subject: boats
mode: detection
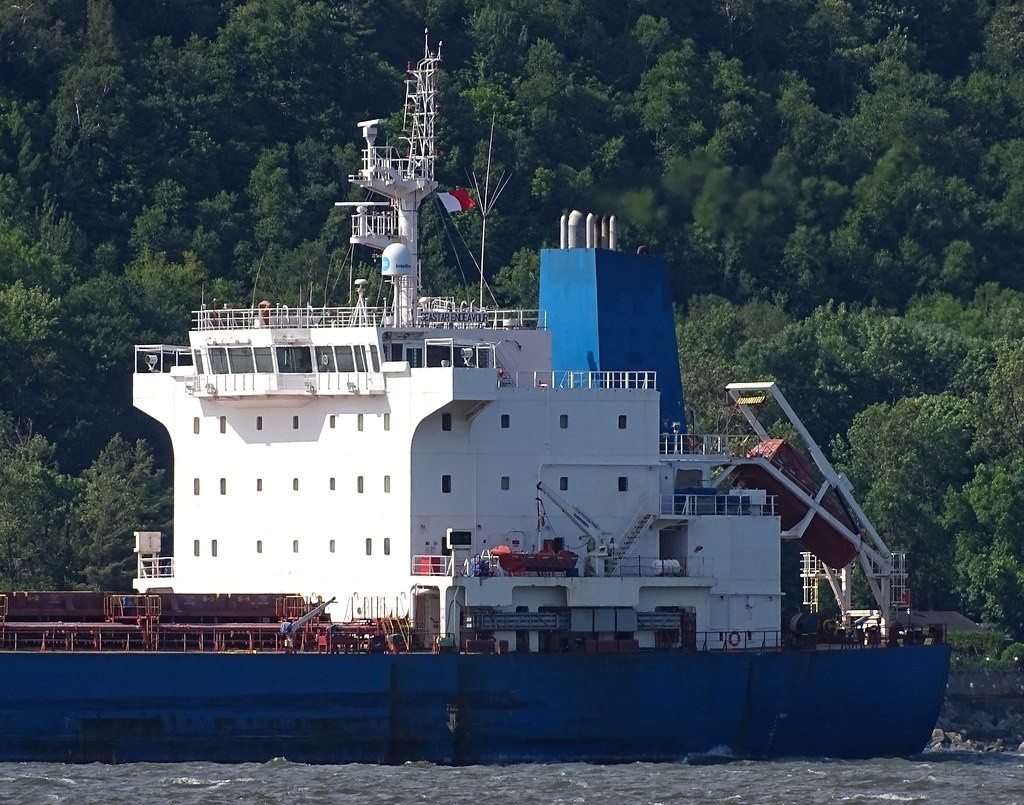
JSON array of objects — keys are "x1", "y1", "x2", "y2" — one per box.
[
  {"x1": 490, "y1": 546, "x2": 579, "y2": 573},
  {"x1": 740, "y1": 440, "x2": 862, "y2": 572},
  {"x1": 1, "y1": 28, "x2": 952, "y2": 768}
]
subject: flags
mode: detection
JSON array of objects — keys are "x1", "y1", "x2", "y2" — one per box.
[{"x1": 437, "y1": 190, "x2": 476, "y2": 213}]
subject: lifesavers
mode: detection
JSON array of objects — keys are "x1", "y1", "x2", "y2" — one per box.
[
  {"x1": 686, "y1": 435, "x2": 700, "y2": 448},
  {"x1": 729, "y1": 631, "x2": 740, "y2": 646},
  {"x1": 823, "y1": 619, "x2": 836, "y2": 634}
]
